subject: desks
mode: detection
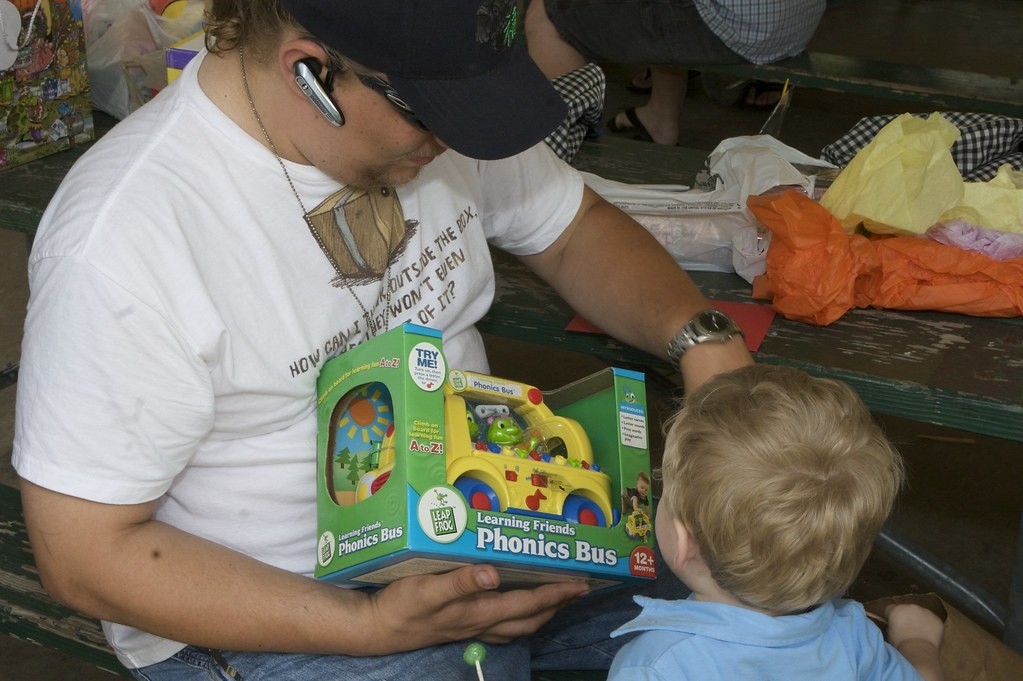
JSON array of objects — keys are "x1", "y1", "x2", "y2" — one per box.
[{"x1": 0, "y1": 129, "x2": 1022, "y2": 628}]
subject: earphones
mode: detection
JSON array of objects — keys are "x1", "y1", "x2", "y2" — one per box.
[{"x1": 293, "y1": 55, "x2": 345, "y2": 128}]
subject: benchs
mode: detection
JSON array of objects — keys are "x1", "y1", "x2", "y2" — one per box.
[
  {"x1": 0, "y1": 478, "x2": 132, "y2": 681},
  {"x1": 641, "y1": 51, "x2": 1022, "y2": 121}
]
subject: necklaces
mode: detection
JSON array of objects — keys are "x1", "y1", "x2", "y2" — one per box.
[{"x1": 237, "y1": 43, "x2": 398, "y2": 338}]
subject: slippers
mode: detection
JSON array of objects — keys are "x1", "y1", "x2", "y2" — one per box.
[
  {"x1": 732, "y1": 80, "x2": 791, "y2": 109},
  {"x1": 606, "y1": 107, "x2": 654, "y2": 143},
  {"x1": 639, "y1": 68, "x2": 652, "y2": 95}
]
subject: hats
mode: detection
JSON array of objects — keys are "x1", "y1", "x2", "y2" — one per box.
[{"x1": 286, "y1": 0, "x2": 568, "y2": 160}]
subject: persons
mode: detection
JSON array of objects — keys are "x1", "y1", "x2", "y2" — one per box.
[
  {"x1": 11, "y1": 0, "x2": 757, "y2": 679},
  {"x1": 522, "y1": 0, "x2": 826, "y2": 147},
  {"x1": 605, "y1": 363, "x2": 945, "y2": 681}
]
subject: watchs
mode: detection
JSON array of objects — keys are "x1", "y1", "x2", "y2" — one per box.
[{"x1": 666, "y1": 308, "x2": 748, "y2": 375}]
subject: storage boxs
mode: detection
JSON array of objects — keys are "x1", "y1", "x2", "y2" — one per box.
[
  {"x1": 0, "y1": 0, "x2": 93, "y2": 172},
  {"x1": 314, "y1": 322, "x2": 659, "y2": 591},
  {"x1": 164, "y1": 25, "x2": 210, "y2": 83}
]
